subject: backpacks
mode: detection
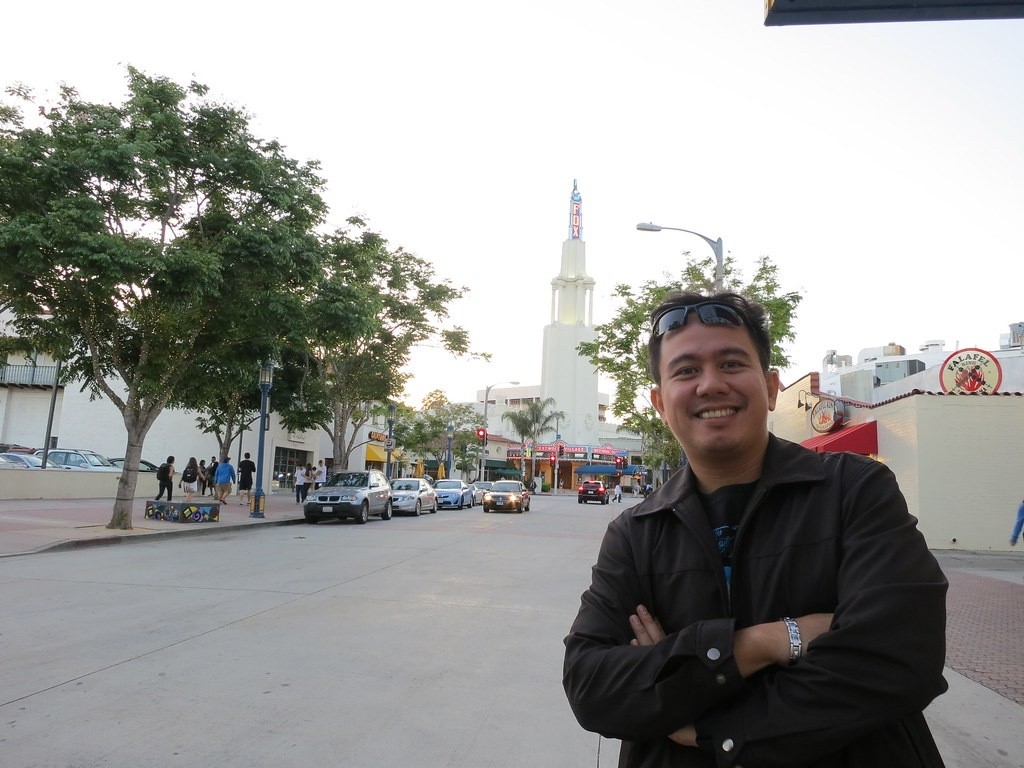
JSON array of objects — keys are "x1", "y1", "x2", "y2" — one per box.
[
  {"x1": 183, "y1": 466, "x2": 197, "y2": 483},
  {"x1": 157, "y1": 464, "x2": 171, "y2": 481}
]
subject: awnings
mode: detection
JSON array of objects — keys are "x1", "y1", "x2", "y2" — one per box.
[
  {"x1": 486, "y1": 460, "x2": 507, "y2": 467},
  {"x1": 366, "y1": 444, "x2": 394, "y2": 462},
  {"x1": 799, "y1": 420, "x2": 878, "y2": 454},
  {"x1": 415, "y1": 460, "x2": 447, "y2": 468}
]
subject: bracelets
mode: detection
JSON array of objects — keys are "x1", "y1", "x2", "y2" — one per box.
[{"x1": 780, "y1": 617, "x2": 802, "y2": 660}]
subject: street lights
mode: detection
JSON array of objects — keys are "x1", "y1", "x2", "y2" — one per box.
[
  {"x1": 480, "y1": 382, "x2": 521, "y2": 482},
  {"x1": 634, "y1": 222, "x2": 725, "y2": 294},
  {"x1": 248, "y1": 358, "x2": 273, "y2": 518},
  {"x1": 385, "y1": 405, "x2": 396, "y2": 480},
  {"x1": 446, "y1": 421, "x2": 454, "y2": 479}
]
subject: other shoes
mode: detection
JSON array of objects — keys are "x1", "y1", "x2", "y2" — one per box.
[{"x1": 221, "y1": 500, "x2": 227, "y2": 505}]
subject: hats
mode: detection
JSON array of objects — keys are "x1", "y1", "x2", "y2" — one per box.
[{"x1": 223, "y1": 457, "x2": 231, "y2": 463}]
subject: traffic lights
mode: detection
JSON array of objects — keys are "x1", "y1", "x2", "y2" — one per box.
[
  {"x1": 615, "y1": 457, "x2": 623, "y2": 470},
  {"x1": 617, "y1": 472, "x2": 621, "y2": 477},
  {"x1": 549, "y1": 454, "x2": 556, "y2": 467},
  {"x1": 621, "y1": 457, "x2": 629, "y2": 469},
  {"x1": 559, "y1": 445, "x2": 564, "y2": 456},
  {"x1": 558, "y1": 469, "x2": 562, "y2": 474},
  {"x1": 477, "y1": 428, "x2": 488, "y2": 446}
]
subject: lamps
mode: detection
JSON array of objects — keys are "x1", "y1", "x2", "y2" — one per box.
[
  {"x1": 804, "y1": 392, "x2": 818, "y2": 411},
  {"x1": 797, "y1": 390, "x2": 814, "y2": 408}
]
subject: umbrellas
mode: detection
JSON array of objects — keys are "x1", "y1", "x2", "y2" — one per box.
[
  {"x1": 437, "y1": 462, "x2": 445, "y2": 480},
  {"x1": 414, "y1": 457, "x2": 425, "y2": 478},
  {"x1": 495, "y1": 468, "x2": 520, "y2": 480}
]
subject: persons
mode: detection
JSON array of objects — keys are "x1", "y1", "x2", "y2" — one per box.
[
  {"x1": 295, "y1": 460, "x2": 326, "y2": 505},
  {"x1": 1009, "y1": 501, "x2": 1024, "y2": 546},
  {"x1": 178, "y1": 457, "x2": 206, "y2": 501},
  {"x1": 238, "y1": 452, "x2": 256, "y2": 506},
  {"x1": 633, "y1": 482, "x2": 641, "y2": 498},
  {"x1": 198, "y1": 457, "x2": 219, "y2": 496},
  {"x1": 214, "y1": 457, "x2": 236, "y2": 506},
  {"x1": 155, "y1": 456, "x2": 175, "y2": 501},
  {"x1": 563, "y1": 291, "x2": 949, "y2": 768},
  {"x1": 612, "y1": 482, "x2": 622, "y2": 503},
  {"x1": 646, "y1": 483, "x2": 652, "y2": 491}
]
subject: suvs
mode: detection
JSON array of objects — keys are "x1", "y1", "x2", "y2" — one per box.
[
  {"x1": 302, "y1": 467, "x2": 393, "y2": 524},
  {"x1": 577, "y1": 480, "x2": 610, "y2": 505}
]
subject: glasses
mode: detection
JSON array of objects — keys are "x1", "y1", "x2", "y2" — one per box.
[{"x1": 653, "y1": 301, "x2": 759, "y2": 342}]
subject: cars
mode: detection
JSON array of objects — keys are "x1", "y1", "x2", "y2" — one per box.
[
  {"x1": 431, "y1": 479, "x2": 473, "y2": 510},
  {"x1": 482, "y1": 479, "x2": 531, "y2": 514},
  {"x1": 0, "y1": 442, "x2": 123, "y2": 471},
  {"x1": 108, "y1": 457, "x2": 159, "y2": 472},
  {"x1": 388, "y1": 477, "x2": 438, "y2": 516},
  {"x1": 469, "y1": 481, "x2": 495, "y2": 506}
]
